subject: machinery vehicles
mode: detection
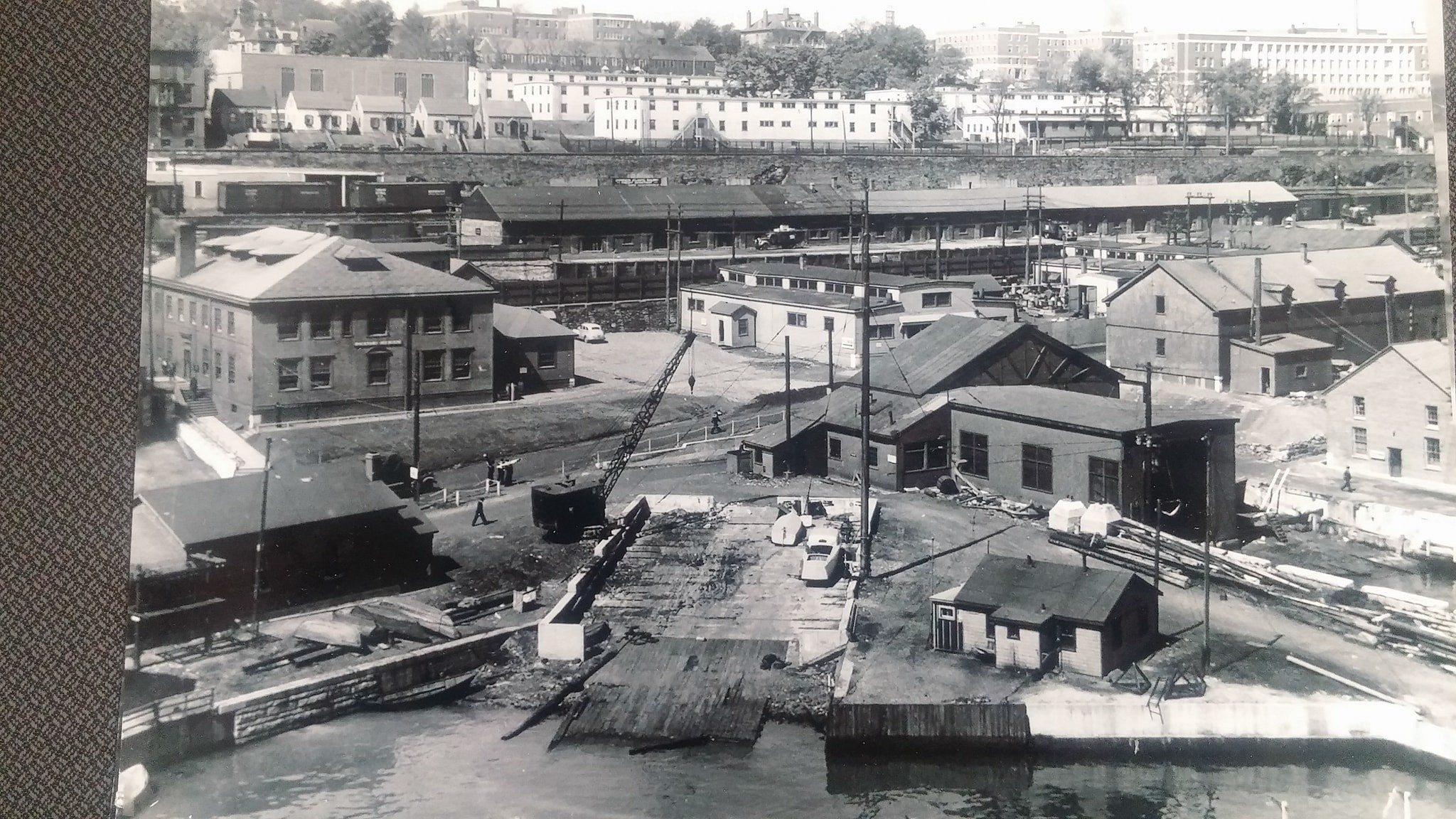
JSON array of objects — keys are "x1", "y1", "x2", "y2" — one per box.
[{"x1": 531, "y1": 330, "x2": 697, "y2": 535}]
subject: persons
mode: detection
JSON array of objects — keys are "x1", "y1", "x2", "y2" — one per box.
[
  {"x1": 1341, "y1": 467, "x2": 1352, "y2": 492},
  {"x1": 472, "y1": 497, "x2": 489, "y2": 526},
  {"x1": 711, "y1": 411, "x2": 722, "y2": 434},
  {"x1": 486, "y1": 459, "x2": 513, "y2": 487}
]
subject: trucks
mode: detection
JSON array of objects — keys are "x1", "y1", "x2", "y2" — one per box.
[
  {"x1": 754, "y1": 229, "x2": 806, "y2": 250},
  {"x1": 799, "y1": 527, "x2": 843, "y2": 580},
  {"x1": 379, "y1": 453, "x2": 437, "y2": 497}
]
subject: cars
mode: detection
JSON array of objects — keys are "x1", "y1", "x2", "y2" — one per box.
[{"x1": 572, "y1": 322, "x2": 605, "y2": 343}]
